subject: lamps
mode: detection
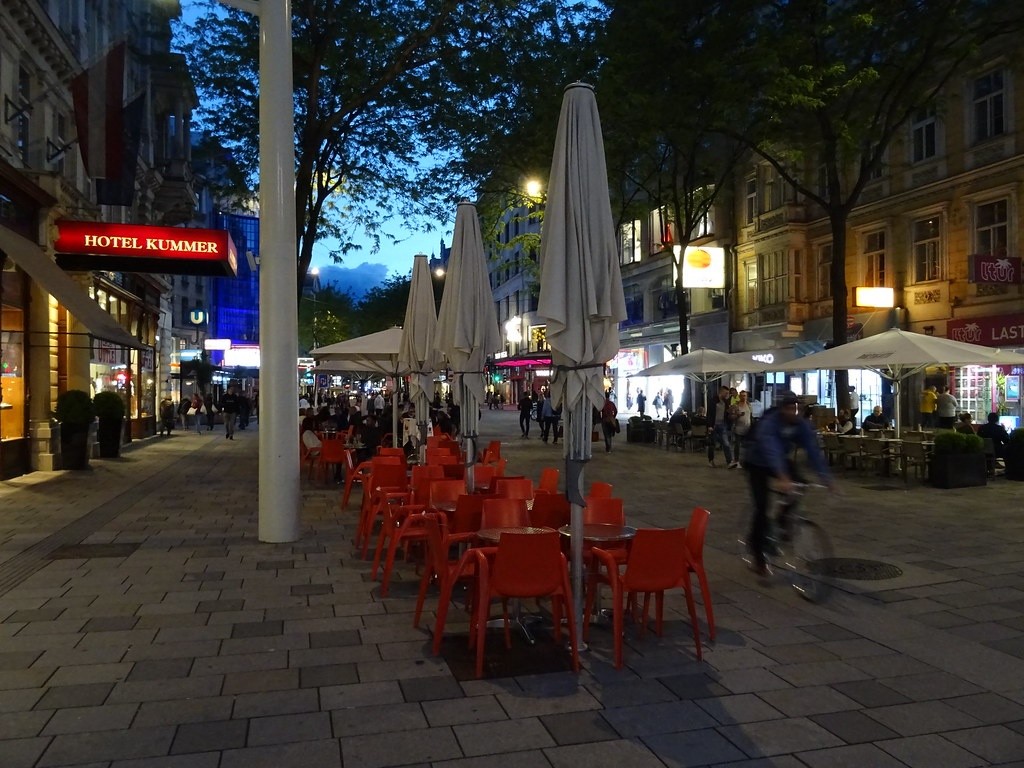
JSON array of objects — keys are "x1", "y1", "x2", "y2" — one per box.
[{"x1": 852, "y1": 286, "x2": 894, "y2": 308}]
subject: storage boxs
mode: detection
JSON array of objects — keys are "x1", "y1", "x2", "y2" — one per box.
[{"x1": 798, "y1": 395, "x2": 835, "y2": 427}]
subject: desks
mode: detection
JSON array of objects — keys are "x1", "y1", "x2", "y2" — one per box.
[
  {"x1": 869, "y1": 428, "x2": 895, "y2": 438},
  {"x1": 524, "y1": 499, "x2": 535, "y2": 510},
  {"x1": 816, "y1": 431, "x2": 843, "y2": 468},
  {"x1": 555, "y1": 522, "x2": 636, "y2": 634},
  {"x1": 903, "y1": 439, "x2": 935, "y2": 485},
  {"x1": 875, "y1": 437, "x2": 903, "y2": 479},
  {"x1": 908, "y1": 430, "x2": 934, "y2": 439},
  {"x1": 476, "y1": 529, "x2": 554, "y2": 645},
  {"x1": 430, "y1": 499, "x2": 469, "y2": 591},
  {"x1": 839, "y1": 435, "x2": 868, "y2": 472}
]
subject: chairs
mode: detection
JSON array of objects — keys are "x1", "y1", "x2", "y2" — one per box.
[
  {"x1": 804, "y1": 427, "x2": 1006, "y2": 485},
  {"x1": 653, "y1": 419, "x2": 708, "y2": 453},
  {"x1": 301, "y1": 430, "x2": 715, "y2": 679}
]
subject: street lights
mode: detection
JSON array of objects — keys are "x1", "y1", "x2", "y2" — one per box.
[{"x1": 311, "y1": 267, "x2": 320, "y2": 351}]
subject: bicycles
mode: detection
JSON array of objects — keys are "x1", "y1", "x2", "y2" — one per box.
[{"x1": 738, "y1": 482, "x2": 834, "y2": 603}]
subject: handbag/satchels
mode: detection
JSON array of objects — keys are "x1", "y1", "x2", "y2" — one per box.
[
  {"x1": 187, "y1": 407, "x2": 196, "y2": 415},
  {"x1": 201, "y1": 404, "x2": 207, "y2": 415},
  {"x1": 211, "y1": 403, "x2": 218, "y2": 413},
  {"x1": 606, "y1": 415, "x2": 617, "y2": 428}
]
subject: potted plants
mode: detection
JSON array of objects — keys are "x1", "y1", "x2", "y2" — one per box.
[
  {"x1": 626, "y1": 415, "x2": 655, "y2": 443},
  {"x1": 1002, "y1": 428, "x2": 1024, "y2": 481},
  {"x1": 928, "y1": 428, "x2": 988, "y2": 488},
  {"x1": 93, "y1": 390, "x2": 126, "y2": 459},
  {"x1": 56, "y1": 390, "x2": 96, "y2": 469}
]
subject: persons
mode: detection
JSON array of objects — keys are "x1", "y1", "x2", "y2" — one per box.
[
  {"x1": 190, "y1": 383, "x2": 260, "y2": 441},
  {"x1": 299, "y1": 385, "x2": 499, "y2": 485},
  {"x1": 516, "y1": 384, "x2": 1008, "y2": 567}
]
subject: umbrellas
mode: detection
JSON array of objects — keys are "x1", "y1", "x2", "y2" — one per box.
[
  {"x1": 625, "y1": 346, "x2": 773, "y2": 417},
  {"x1": 398, "y1": 254, "x2": 448, "y2": 446},
  {"x1": 770, "y1": 327, "x2": 1024, "y2": 471},
  {"x1": 307, "y1": 323, "x2": 451, "y2": 450},
  {"x1": 431, "y1": 198, "x2": 504, "y2": 492},
  {"x1": 308, "y1": 360, "x2": 398, "y2": 379},
  {"x1": 534, "y1": 79, "x2": 628, "y2": 653}
]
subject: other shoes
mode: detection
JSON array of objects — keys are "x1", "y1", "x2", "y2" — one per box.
[
  {"x1": 552, "y1": 441, "x2": 557, "y2": 444},
  {"x1": 226, "y1": 433, "x2": 230, "y2": 439},
  {"x1": 230, "y1": 436, "x2": 233, "y2": 440},
  {"x1": 207, "y1": 427, "x2": 210, "y2": 431},
  {"x1": 726, "y1": 463, "x2": 737, "y2": 469},
  {"x1": 709, "y1": 461, "x2": 716, "y2": 468},
  {"x1": 211, "y1": 427, "x2": 214, "y2": 430},
  {"x1": 751, "y1": 553, "x2": 774, "y2": 578},
  {"x1": 543, "y1": 438, "x2": 546, "y2": 442},
  {"x1": 522, "y1": 433, "x2": 527, "y2": 440}
]
